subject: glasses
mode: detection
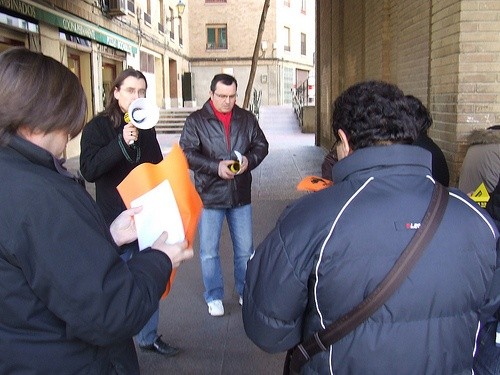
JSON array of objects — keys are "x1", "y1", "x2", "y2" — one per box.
[
  {"x1": 329, "y1": 139, "x2": 339, "y2": 158},
  {"x1": 214, "y1": 91, "x2": 238, "y2": 101},
  {"x1": 119, "y1": 87, "x2": 146, "y2": 96}
]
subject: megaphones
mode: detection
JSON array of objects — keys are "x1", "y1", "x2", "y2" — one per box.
[{"x1": 123, "y1": 98, "x2": 160, "y2": 147}]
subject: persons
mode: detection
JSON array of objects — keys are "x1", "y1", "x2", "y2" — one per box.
[
  {"x1": 79, "y1": 67, "x2": 183, "y2": 355},
  {"x1": 0, "y1": 46, "x2": 194, "y2": 375},
  {"x1": 179, "y1": 74, "x2": 269, "y2": 316},
  {"x1": 243, "y1": 81, "x2": 499, "y2": 375}
]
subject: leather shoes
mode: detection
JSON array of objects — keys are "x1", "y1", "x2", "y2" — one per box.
[{"x1": 139, "y1": 335, "x2": 180, "y2": 357}]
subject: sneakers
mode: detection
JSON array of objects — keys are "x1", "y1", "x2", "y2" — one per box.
[
  {"x1": 207, "y1": 299, "x2": 224, "y2": 316},
  {"x1": 238, "y1": 297, "x2": 243, "y2": 306}
]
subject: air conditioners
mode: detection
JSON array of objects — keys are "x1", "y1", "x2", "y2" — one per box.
[{"x1": 108, "y1": 0, "x2": 128, "y2": 15}]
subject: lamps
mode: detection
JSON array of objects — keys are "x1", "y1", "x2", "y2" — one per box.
[{"x1": 166, "y1": 0, "x2": 185, "y2": 24}]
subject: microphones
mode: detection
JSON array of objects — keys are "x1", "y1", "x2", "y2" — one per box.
[{"x1": 231, "y1": 162, "x2": 240, "y2": 174}]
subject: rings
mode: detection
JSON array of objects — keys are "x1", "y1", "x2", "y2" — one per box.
[{"x1": 131, "y1": 131, "x2": 133, "y2": 136}]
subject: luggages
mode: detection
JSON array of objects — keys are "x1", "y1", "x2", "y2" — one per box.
[{"x1": 322, "y1": 154, "x2": 338, "y2": 181}]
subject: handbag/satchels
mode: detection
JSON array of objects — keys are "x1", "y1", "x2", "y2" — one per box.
[{"x1": 283, "y1": 349, "x2": 305, "y2": 375}]
